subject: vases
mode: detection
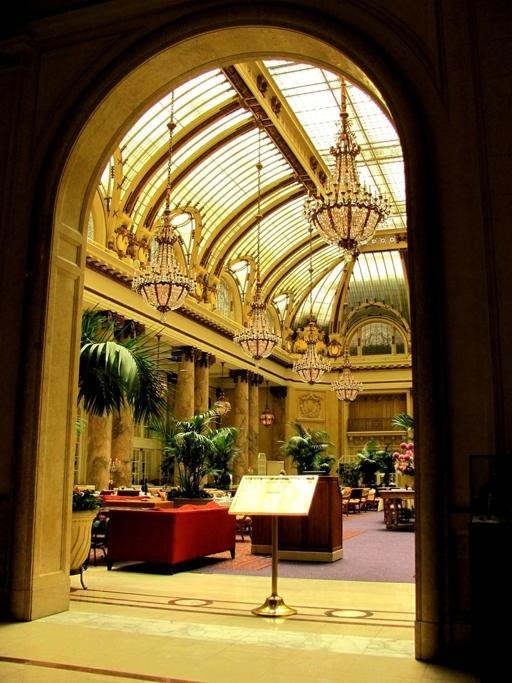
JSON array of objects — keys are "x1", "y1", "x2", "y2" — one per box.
[
  {"x1": 70, "y1": 507, "x2": 100, "y2": 571},
  {"x1": 398, "y1": 475, "x2": 415, "y2": 490}
]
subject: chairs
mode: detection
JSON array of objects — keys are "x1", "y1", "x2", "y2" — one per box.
[{"x1": 97, "y1": 486, "x2": 379, "y2": 576}]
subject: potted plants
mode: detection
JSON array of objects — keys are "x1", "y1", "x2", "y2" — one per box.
[{"x1": 144, "y1": 405, "x2": 240, "y2": 506}]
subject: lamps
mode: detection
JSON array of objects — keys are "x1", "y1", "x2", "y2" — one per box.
[
  {"x1": 150, "y1": 334, "x2": 168, "y2": 402},
  {"x1": 330, "y1": 277, "x2": 362, "y2": 402},
  {"x1": 260, "y1": 381, "x2": 275, "y2": 429},
  {"x1": 131, "y1": 90, "x2": 195, "y2": 313},
  {"x1": 292, "y1": 192, "x2": 329, "y2": 384},
  {"x1": 214, "y1": 361, "x2": 231, "y2": 417},
  {"x1": 233, "y1": 128, "x2": 283, "y2": 359},
  {"x1": 301, "y1": 76, "x2": 391, "y2": 262}
]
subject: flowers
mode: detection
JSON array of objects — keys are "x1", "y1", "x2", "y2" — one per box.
[
  {"x1": 72, "y1": 485, "x2": 101, "y2": 512},
  {"x1": 391, "y1": 442, "x2": 416, "y2": 475}
]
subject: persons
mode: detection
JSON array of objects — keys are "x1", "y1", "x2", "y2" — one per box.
[
  {"x1": 341, "y1": 469, "x2": 396, "y2": 510},
  {"x1": 279, "y1": 469, "x2": 286, "y2": 475}
]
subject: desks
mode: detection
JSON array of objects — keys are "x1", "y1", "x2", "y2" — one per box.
[{"x1": 378, "y1": 490, "x2": 416, "y2": 531}]
